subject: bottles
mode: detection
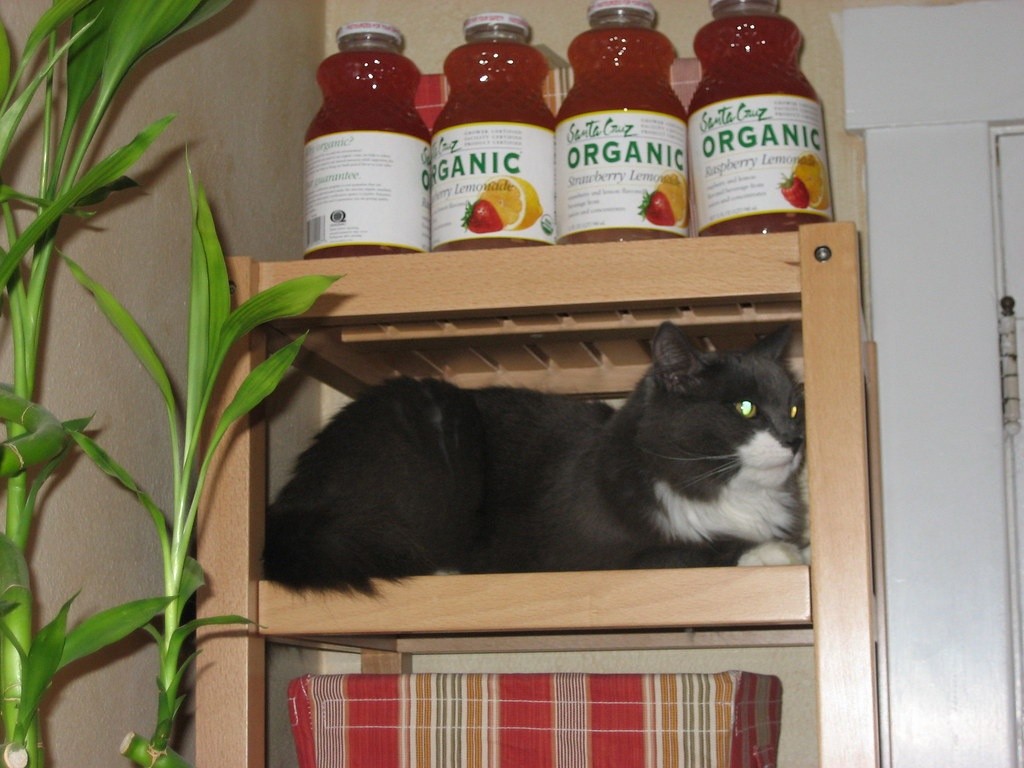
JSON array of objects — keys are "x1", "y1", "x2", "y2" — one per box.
[
  {"x1": 300, "y1": 21, "x2": 431, "y2": 262},
  {"x1": 687, "y1": 0, "x2": 837, "y2": 238},
  {"x1": 430, "y1": 9, "x2": 554, "y2": 251},
  {"x1": 555, "y1": 1, "x2": 690, "y2": 241}
]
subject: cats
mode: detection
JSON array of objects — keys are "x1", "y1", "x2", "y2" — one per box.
[{"x1": 260, "y1": 319, "x2": 813, "y2": 601}]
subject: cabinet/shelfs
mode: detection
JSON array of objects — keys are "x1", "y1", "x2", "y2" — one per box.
[{"x1": 190, "y1": 222, "x2": 881, "y2": 768}]
